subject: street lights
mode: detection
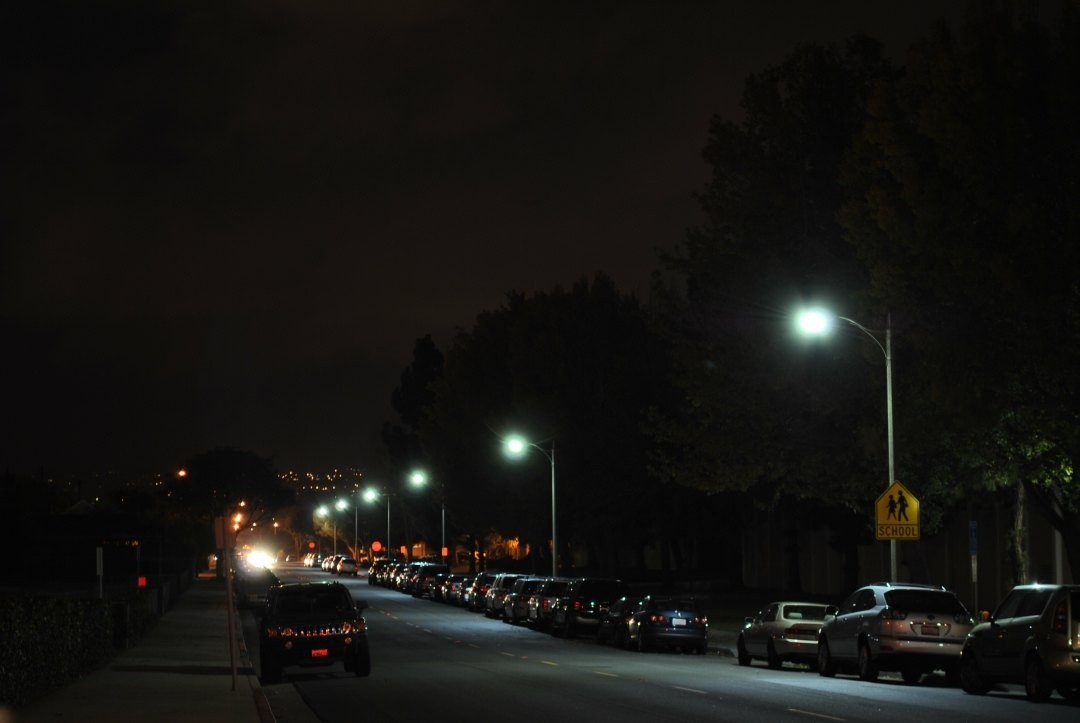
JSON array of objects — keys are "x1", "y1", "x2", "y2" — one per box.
[
  {"x1": 366, "y1": 491, "x2": 391, "y2": 559},
  {"x1": 340, "y1": 500, "x2": 358, "y2": 555},
  {"x1": 509, "y1": 438, "x2": 559, "y2": 578},
  {"x1": 414, "y1": 474, "x2": 447, "y2": 565},
  {"x1": 799, "y1": 306, "x2": 898, "y2": 581},
  {"x1": 318, "y1": 504, "x2": 337, "y2": 556}
]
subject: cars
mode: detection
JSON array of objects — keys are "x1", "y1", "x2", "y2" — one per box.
[
  {"x1": 959, "y1": 582, "x2": 1080, "y2": 705},
  {"x1": 736, "y1": 602, "x2": 839, "y2": 669},
  {"x1": 231, "y1": 557, "x2": 283, "y2": 606},
  {"x1": 302, "y1": 552, "x2": 358, "y2": 577},
  {"x1": 260, "y1": 581, "x2": 370, "y2": 672},
  {"x1": 367, "y1": 559, "x2": 710, "y2": 656}
]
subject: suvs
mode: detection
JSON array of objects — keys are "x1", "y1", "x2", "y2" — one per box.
[{"x1": 817, "y1": 581, "x2": 978, "y2": 684}]
